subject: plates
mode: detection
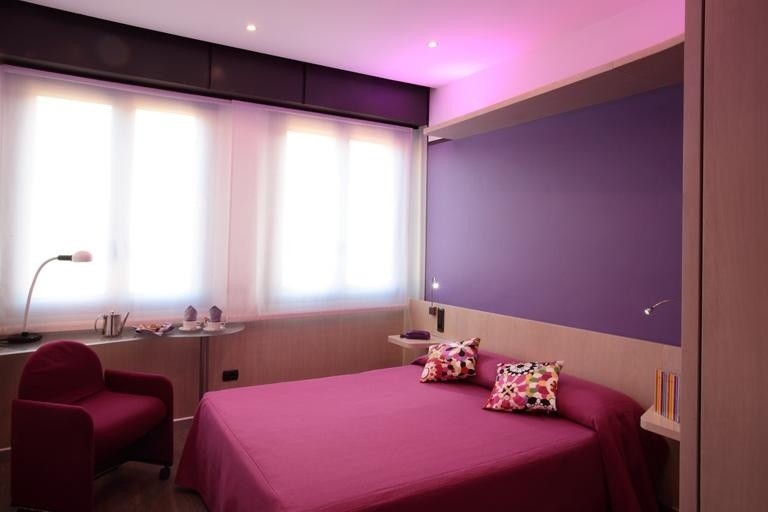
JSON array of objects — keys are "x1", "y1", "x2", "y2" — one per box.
[
  {"x1": 178, "y1": 326, "x2": 201, "y2": 331},
  {"x1": 130, "y1": 321, "x2": 174, "y2": 334},
  {"x1": 203, "y1": 326, "x2": 226, "y2": 331}
]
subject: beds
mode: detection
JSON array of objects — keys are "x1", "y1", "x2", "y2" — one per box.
[{"x1": 193, "y1": 344, "x2": 656, "y2": 511}]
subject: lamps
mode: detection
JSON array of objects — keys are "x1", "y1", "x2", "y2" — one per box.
[
  {"x1": 6, "y1": 250, "x2": 94, "y2": 345},
  {"x1": 428, "y1": 276, "x2": 440, "y2": 316},
  {"x1": 643, "y1": 297, "x2": 681, "y2": 316}
]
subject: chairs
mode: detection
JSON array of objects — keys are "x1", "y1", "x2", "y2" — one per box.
[{"x1": 11, "y1": 340, "x2": 174, "y2": 512}]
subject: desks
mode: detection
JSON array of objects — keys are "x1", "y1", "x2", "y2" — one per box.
[
  {"x1": 387, "y1": 334, "x2": 440, "y2": 366},
  {"x1": 640, "y1": 402, "x2": 680, "y2": 511},
  {"x1": 0, "y1": 319, "x2": 246, "y2": 402}
]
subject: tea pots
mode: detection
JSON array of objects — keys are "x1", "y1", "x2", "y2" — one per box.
[{"x1": 94, "y1": 311, "x2": 130, "y2": 336}]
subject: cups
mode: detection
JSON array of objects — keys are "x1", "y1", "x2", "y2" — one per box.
[
  {"x1": 183, "y1": 321, "x2": 201, "y2": 330},
  {"x1": 207, "y1": 321, "x2": 225, "y2": 329}
]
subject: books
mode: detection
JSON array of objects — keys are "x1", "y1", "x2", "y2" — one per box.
[{"x1": 655, "y1": 369, "x2": 680, "y2": 423}]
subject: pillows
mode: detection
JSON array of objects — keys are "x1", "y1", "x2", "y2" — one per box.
[{"x1": 419, "y1": 335, "x2": 564, "y2": 414}]
